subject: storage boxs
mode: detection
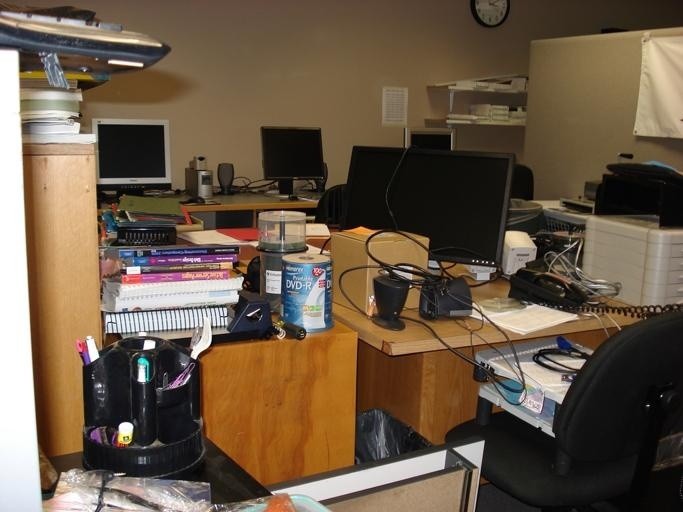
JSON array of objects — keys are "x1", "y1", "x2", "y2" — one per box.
[{"x1": 329, "y1": 225, "x2": 430, "y2": 318}]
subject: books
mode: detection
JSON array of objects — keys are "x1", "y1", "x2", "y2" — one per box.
[
  {"x1": 15, "y1": 66, "x2": 111, "y2": 146},
  {"x1": 471, "y1": 337, "x2": 601, "y2": 438},
  {"x1": 91, "y1": 194, "x2": 261, "y2": 340},
  {"x1": 275, "y1": 222, "x2": 330, "y2": 241}
]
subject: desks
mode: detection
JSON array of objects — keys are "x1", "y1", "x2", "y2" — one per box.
[
  {"x1": 40, "y1": 435, "x2": 275, "y2": 511},
  {"x1": 159, "y1": 190, "x2": 319, "y2": 229},
  {"x1": 177, "y1": 227, "x2": 648, "y2": 446}
]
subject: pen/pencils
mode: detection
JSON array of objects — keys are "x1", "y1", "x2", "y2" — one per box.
[
  {"x1": 76, "y1": 335, "x2": 100, "y2": 366},
  {"x1": 136, "y1": 358, "x2": 150, "y2": 383}
]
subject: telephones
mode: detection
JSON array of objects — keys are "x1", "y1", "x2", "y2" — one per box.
[{"x1": 508, "y1": 267, "x2": 594, "y2": 314}]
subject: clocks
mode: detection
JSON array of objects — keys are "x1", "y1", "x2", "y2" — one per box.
[{"x1": 467, "y1": 0, "x2": 512, "y2": 29}]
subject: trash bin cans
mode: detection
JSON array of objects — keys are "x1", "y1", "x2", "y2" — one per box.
[{"x1": 355, "y1": 407, "x2": 433, "y2": 465}]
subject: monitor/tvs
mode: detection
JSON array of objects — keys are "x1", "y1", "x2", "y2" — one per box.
[
  {"x1": 340, "y1": 145, "x2": 516, "y2": 267},
  {"x1": 403, "y1": 127, "x2": 456, "y2": 151},
  {"x1": 260, "y1": 125, "x2": 325, "y2": 199},
  {"x1": 92, "y1": 117, "x2": 172, "y2": 197}
]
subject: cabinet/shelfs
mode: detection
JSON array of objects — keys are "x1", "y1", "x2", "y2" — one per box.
[
  {"x1": 445, "y1": 85, "x2": 528, "y2": 126},
  {"x1": 194, "y1": 317, "x2": 358, "y2": 487},
  {"x1": 21, "y1": 141, "x2": 107, "y2": 456}
]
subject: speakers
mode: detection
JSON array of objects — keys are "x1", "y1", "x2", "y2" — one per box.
[
  {"x1": 217, "y1": 163, "x2": 235, "y2": 195},
  {"x1": 185, "y1": 168, "x2": 213, "y2": 198},
  {"x1": 308, "y1": 163, "x2": 330, "y2": 192}
]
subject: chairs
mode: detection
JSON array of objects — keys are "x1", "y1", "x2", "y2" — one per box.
[
  {"x1": 509, "y1": 163, "x2": 535, "y2": 201},
  {"x1": 442, "y1": 300, "x2": 683, "y2": 512}
]
